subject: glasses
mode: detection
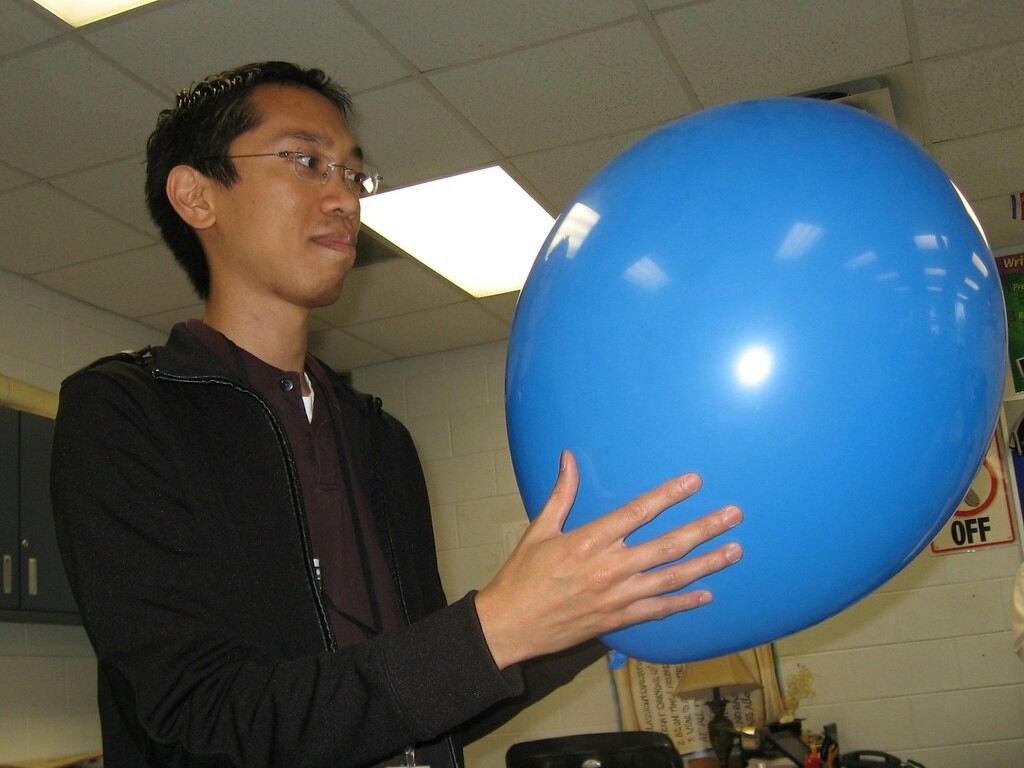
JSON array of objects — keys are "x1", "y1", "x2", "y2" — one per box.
[{"x1": 194, "y1": 150, "x2": 384, "y2": 196}]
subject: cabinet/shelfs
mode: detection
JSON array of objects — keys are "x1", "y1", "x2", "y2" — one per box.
[{"x1": 0, "y1": 373, "x2": 83, "y2": 626}]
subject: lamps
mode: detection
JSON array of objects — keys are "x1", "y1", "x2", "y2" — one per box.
[{"x1": 671, "y1": 652, "x2": 764, "y2": 768}]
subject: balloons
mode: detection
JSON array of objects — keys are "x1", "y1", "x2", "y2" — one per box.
[{"x1": 505, "y1": 95, "x2": 1006, "y2": 672}]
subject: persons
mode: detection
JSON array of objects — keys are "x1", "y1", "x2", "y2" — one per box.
[{"x1": 51, "y1": 61, "x2": 744, "y2": 768}]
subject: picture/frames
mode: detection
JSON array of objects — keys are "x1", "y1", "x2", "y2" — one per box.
[{"x1": 607, "y1": 642, "x2": 785, "y2": 757}]
